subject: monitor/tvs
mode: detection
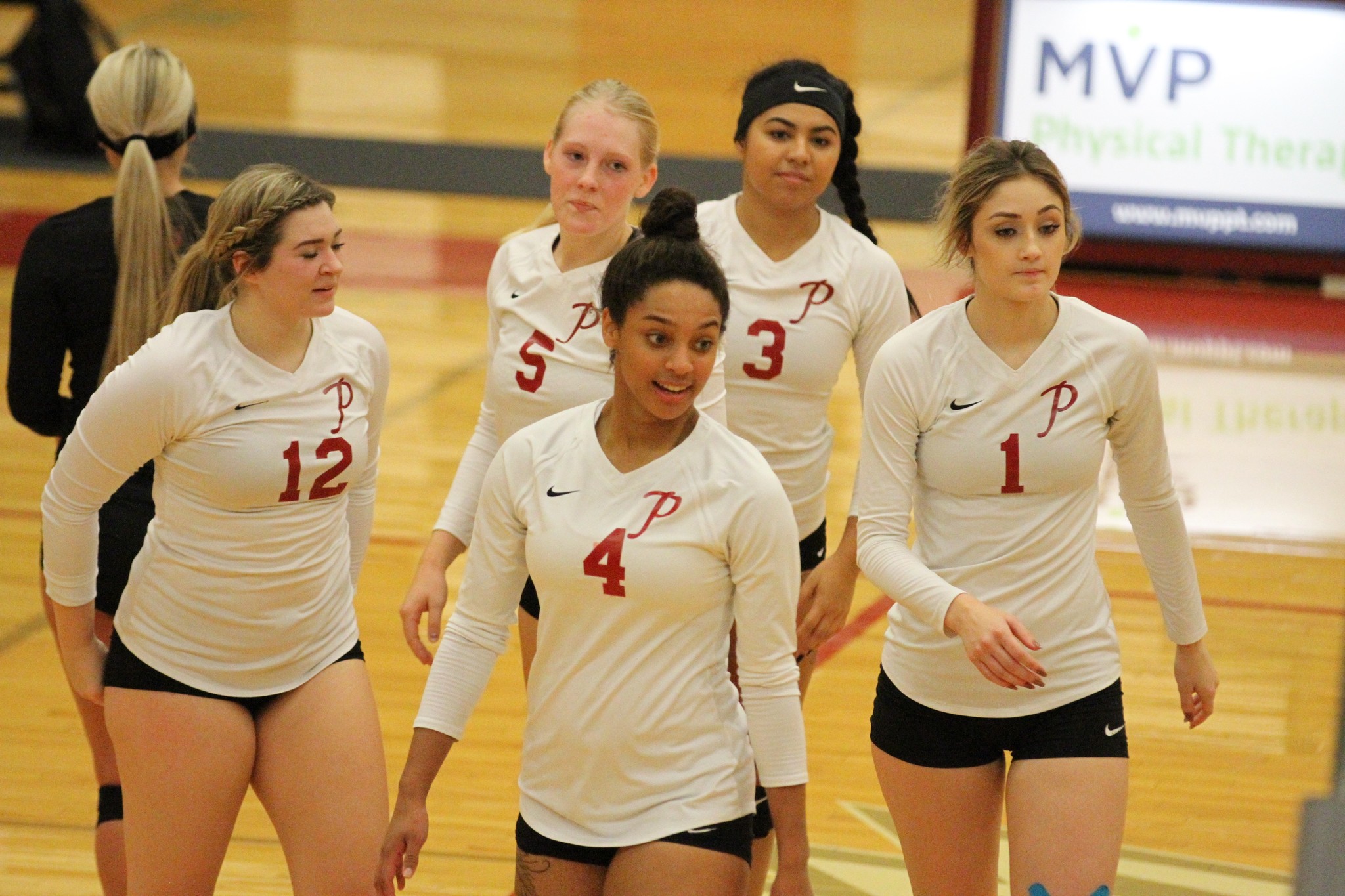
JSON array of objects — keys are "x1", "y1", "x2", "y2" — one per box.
[{"x1": 965, "y1": 0, "x2": 1345, "y2": 283}]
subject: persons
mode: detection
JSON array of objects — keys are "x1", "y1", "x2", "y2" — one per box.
[
  {"x1": 373, "y1": 186, "x2": 817, "y2": 896},
  {"x1": 36, "y1": 162, "x2": 396, "y2": 896},
  {"x1": 397, "y1": 81, "x2": 734, "y2": 691},
  {"x1": 6, "y1": 38, "x2": 238, "y2": 896},
  {"x1": 691, "y1": 58, "x2": 927, "y2": 896},
  {"x1": 854, "y1": 137, "x2": 1221, "y2": 896}
]
